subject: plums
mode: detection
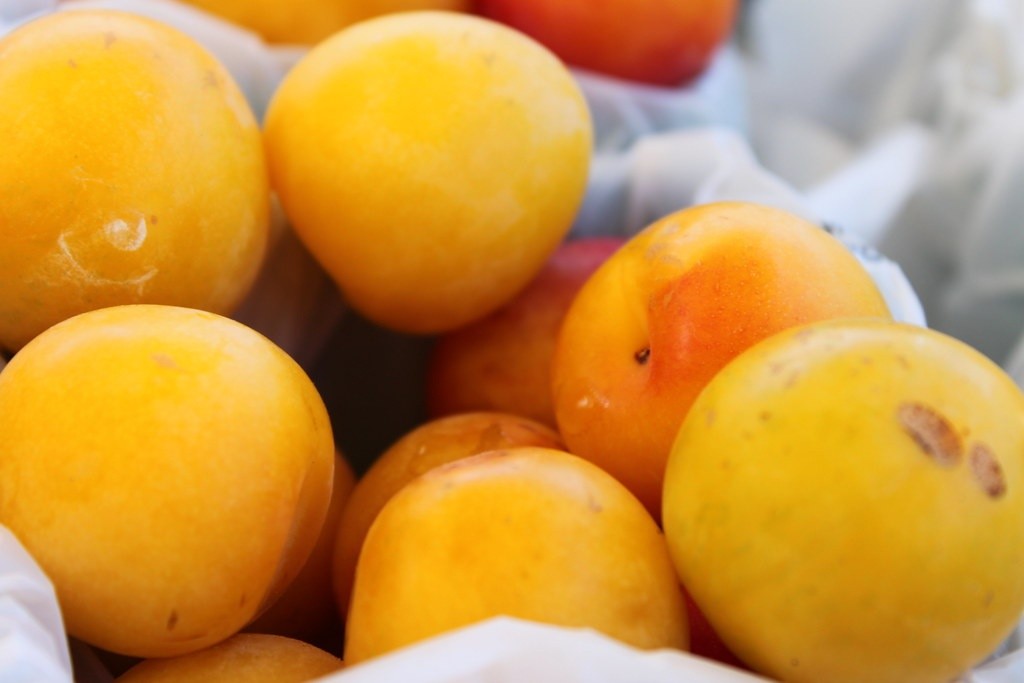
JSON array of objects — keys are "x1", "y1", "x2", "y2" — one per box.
[{"x1": 1, "y1": 8, "x2": 1024, "y2": 683}]
